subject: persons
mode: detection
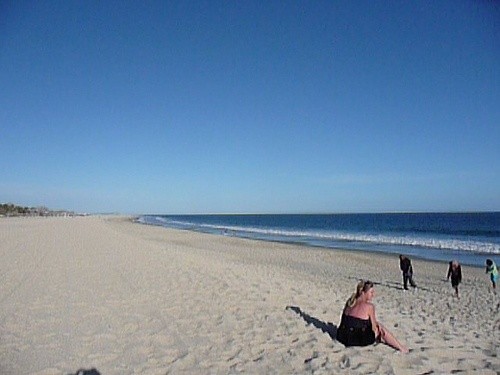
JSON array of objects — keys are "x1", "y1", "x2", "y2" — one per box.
[
  {"x1": 336, "y1": 278, "x2": 411, "y2": 354},
  {"x1": 399, "y1": 254, "x2": 418, "y2": 290},
  {"x1": 446, "y1": 260, "x2": 462, "y2": 299},
  {"x1": 484, "y1": 258, "x2": 498, "y2": 288}
]
